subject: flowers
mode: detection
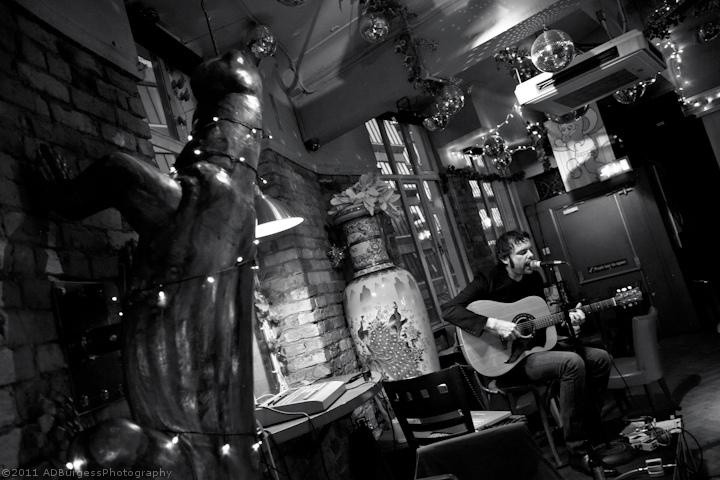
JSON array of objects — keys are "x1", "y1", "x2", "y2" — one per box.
[{"x1": 325, "y1": 169, "x2": 403, "y2": 272}]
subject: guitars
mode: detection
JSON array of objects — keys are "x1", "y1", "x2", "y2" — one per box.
[{"x1": 456, "y1": 285, "x2": 643, "y2": 378}]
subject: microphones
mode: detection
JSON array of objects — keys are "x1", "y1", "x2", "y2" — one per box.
[{"x1": 530, "y1": 259, "x2": 566, "y2": 268}]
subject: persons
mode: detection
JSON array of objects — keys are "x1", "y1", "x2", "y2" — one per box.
[{"x1": 441, "y1": 229, "x2": 618, "y2": 475}]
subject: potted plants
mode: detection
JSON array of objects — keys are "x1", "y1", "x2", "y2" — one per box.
[{"x1": 643, "y1": 0, "x2": 720, "y2": 102}]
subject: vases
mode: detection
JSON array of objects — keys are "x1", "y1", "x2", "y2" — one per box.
[{"x1": 331, "y1": 204, "x2": 445, "y2": 401}]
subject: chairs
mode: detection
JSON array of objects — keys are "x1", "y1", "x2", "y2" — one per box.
[
  {"x1": 609, "y1": 305, "x2": 681, "y2": 415},
  {"x1": 474, "y1": 371, "x2": 562, "y2": 468},
  {"x1": 415, "y1": 422, "x2": 530, "y2": 480},
  {"x1": 382, "y1": 364, "x2": 474, "y2": 446}
]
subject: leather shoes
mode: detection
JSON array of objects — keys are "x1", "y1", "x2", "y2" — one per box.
[{"x1": 568, "y1": 446, "x2": 620, "y2": 478}]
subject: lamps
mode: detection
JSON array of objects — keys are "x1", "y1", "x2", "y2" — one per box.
[
  {"x1": 422, "y1": 107, "x2": 450, "y2": 133},
  {"x1": 482, "y1": 132, "x2": 505, "y2": 159},
  {"x1": 361, "y1": 13, "x2": 390, "y2": 44},
  {"x1": 493, "y1": 150, "x2": 514, "y2": 173},
  {"x1": 433, "y1": 81, "x2": 467, "y2": 117},
  {"x1": 530, "y1": 22, "x2": 576, "y2": 75},
  {"x1": 243, "y1": 19, "x2": 279, "y2": 59},
  {"x1": 254, "y1": 179, "x2": 304, "y2": 239},
  {"x1": 614, "y1": 79, "x2": 649, "y2": 105}
]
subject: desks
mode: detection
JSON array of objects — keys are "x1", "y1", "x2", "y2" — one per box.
[
  {"x1": 252, "y1": 365, "x2": 386, "y2": 479},
  {"x1": 392, "y1": 410, "x2": 512, "y2": 442}
]
